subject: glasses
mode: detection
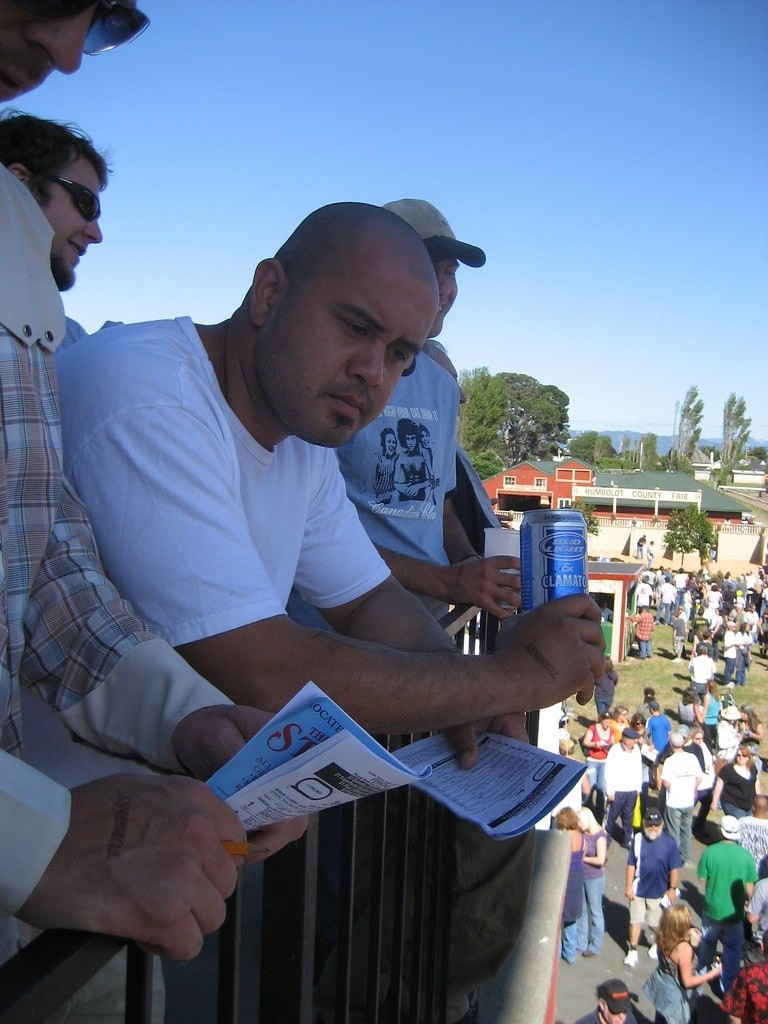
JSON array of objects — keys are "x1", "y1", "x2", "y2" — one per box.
[
  {"x1": 695, "y1": 737, "x2": 704, "y2": 740},
  {"x1": 636, "y1": 723, "x2": 644, "y2": 725},
  {"x1": 24, "y1": 166, "x2": 102, "y2": 221},
  {"x1": 736, "y1": 754, "x2": 747, "y2": 757},
  {"x1": 18, "y1": 0, "x2": 151, "y2": 57}
]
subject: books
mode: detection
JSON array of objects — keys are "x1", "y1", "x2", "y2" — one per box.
[
  {"x1": 134, "y1": 683, "x2": 428, "y2": 865},
  {"x1": 371, "y1": 722, "x2": 594, "y2": 847}
]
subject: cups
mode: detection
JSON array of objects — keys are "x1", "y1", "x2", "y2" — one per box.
[{"x1": 484, "y1": 526, "x2": 521, "y2": 611}]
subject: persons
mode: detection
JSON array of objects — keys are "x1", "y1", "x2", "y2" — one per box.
[
  {"x1": 548, "y1": 567, "x2": 768, "y2": 1024},
  {"x1": 0, "y1": 0, "x2": 605, "y2": 1024},
  {"x1": 636, "y1": 533, "x2": 656, "y2": 569},
  {"x1": 703, "y1": 541, "x2": 716, "y2": 562}
]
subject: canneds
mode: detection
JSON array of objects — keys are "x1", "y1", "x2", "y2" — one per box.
[{"x1": 519, "y1": 507, "x2": 589, "y2": 616}]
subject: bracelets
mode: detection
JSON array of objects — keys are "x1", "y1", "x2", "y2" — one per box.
[
  {"x1": 669, "y1": 886, "x2": 678, "y2": 889},
  {"x1": 455, "y1": 552, "x2": 487, "y2": 567}
]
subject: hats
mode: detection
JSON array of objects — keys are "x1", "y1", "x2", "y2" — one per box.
[
  {"x1": 646, "y1": 701, "x2": 660, "y2": 711},
  {"x1": 644, "y1": 807, "x2": 661, "y2": 826},
  {"x1": 623, "y1": 728, "x2": 639, "y2": 739},
  {"x1": 720, "y1": 815, "x2": 740, "y2": 840},
  {"x1": 676, "y1": 724, "x2": 691, "y2": 738},
  {"x1": 721, "y1": 706, "x2": 741, "y2": 720},
  {"x1": 381, "y1": 198, "x2": 486, "y2": 269},
  {"x1": 598, "y1": 978, "x2": 632, "y2": 1015}
]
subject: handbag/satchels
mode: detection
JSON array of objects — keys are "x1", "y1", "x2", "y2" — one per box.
[
  {"x1": 641, "y1": 744, "x2": 659, "y2": 762},
  {"x1": 660, "y1": 886, "x2": 682, "y2": 911},
  {"x1": 632, "y1": 793, "x2": 641, "y2": 828}
]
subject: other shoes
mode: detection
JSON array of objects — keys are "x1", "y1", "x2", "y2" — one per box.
[
  {"x1": 623, "y1": 947, "x2": 637, "y2": 966},
  {"x1": 648, "y1": 943, "x2": 659, "y2": 960},
  {"x1": 581, "y1": 950, "x2": 594, "y2": 957},
  {"x1": 670, "y1": 656, "x2": 681, "y2": 663},
  {"x1": 723, "y1": 680, "x2": 734, "y2": 689}
]
